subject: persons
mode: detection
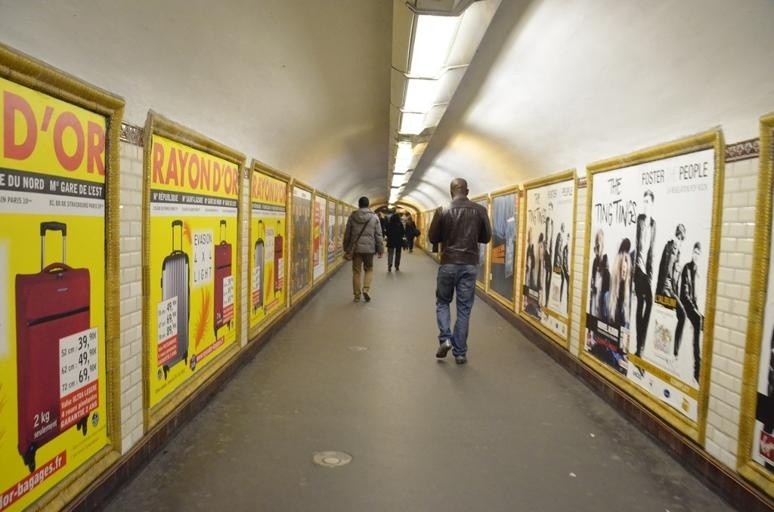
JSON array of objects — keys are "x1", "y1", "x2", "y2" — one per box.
[
  {"x1": 587, "y1": 190, "x2": 704, "y2": 384},
  {"x1": 524, "y1": 216, "x2": 570, "y2": 319},
  {"x1": 427, "y1": 177, "x2": 491, "y2": 365},
  {"x1": 343, "y1": 196, "x2": 383, "y2": 302},
  {"x1": 376, "y1": 211, "x2": 416, "y2": 273}
]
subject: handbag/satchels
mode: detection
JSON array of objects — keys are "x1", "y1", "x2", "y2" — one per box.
[
  {"x1": 414, "y1": 228, "x2": 421, "y2": 237},
  {"x1": 343, "y1": 241, "x2": 356, "y2": 261}
]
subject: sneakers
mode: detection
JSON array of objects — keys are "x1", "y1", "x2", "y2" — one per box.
[
  {"x1": 435, "y1": 337, "x2": 452, "y2": 358},
  {"x1": 362, "y1": 290, "x2": 371, "y2": 302},
  {"x1": 353, "y1": 295, "x2": 360, "y2": 302},
  {"x1": 455, "y1": 354, "x2": 466, "y2": 365}
]
